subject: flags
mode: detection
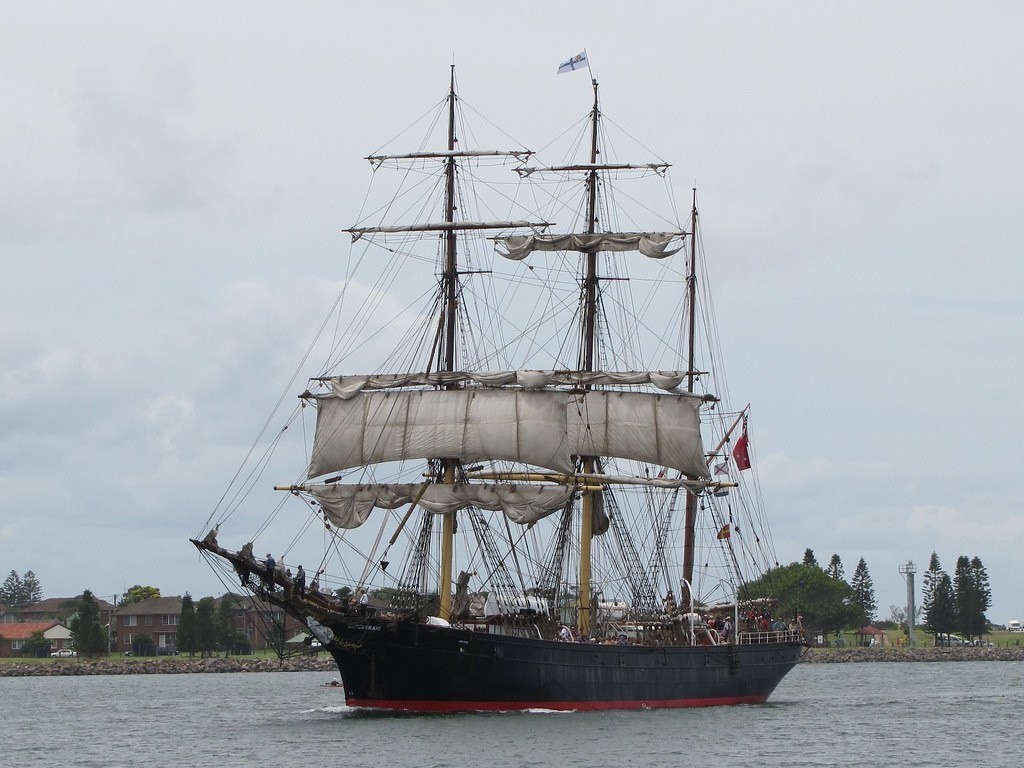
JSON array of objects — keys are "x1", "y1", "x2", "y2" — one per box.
[
  {"x1": 717, "y1": 524, "x2": 730, "y2": 539},
  {"x1": 733, "y1": 415, "x2": 751, "y2": 471},
  {"x1": 557, "y1": 51, "x2": 588, "y2": 74},
  {"x1": 714, "y1": 462, "x2": 728, "y2": 476}
]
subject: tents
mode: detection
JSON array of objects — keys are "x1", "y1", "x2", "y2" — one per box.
[
  {"x1": 286, "y1": 632, "x2": 321, "y2": 657},
  {"x1": 853, "y1": 625, "x2": 885, "y2": 646}
]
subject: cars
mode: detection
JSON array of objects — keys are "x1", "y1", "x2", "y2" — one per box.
[
  {"x1": 124, "y1": 647, "x2": 179, "y2": 656},
  {"x1": 308, "y1": 640, "x2": 321, "y2": 648},
  {"x1": 937, "y1": 634, "x2": 993, "y2": 647},
  {"x1": 50, "y1": 648, "x2": 77, "y2": 658}
]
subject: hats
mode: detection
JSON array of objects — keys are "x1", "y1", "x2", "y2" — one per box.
[
  {"x1": 266, "y1": 553, "x2": 271, "y2": 557},
  {"x1": 297, "y1": 565, "x2": 302, "y2": 569}
]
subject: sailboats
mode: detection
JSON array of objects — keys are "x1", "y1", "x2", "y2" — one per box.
[{"x1": 188, "y1": 63, "x2": 816, "y2": 710}]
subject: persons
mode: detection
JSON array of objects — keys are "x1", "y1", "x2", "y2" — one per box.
[
  {"x1": 258, "y1": 554, "x2": 275, "y2": 592},
  {"x1": 286, "y1": 569, "x2": 292, "y2": 577},
  {"x1": 241, "y1": 550, "x2": 257, "y2": 587},
  {"x1": 294, "y1": 565, "x2": 305, "y2": 600},
  {"x1": 560, "y1": 625, "x2": 640, "y2": 646},
  {"x1": 359, "y1": 590, "x2": 369, "y2": 616},
  {"x1": 704, "y1": 614, "x2": 803, "y2": 642}
]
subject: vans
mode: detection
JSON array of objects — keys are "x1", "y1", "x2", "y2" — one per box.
[{"x1": 1007, "y1": 620, "x2": 1023, "y2": 632}]
